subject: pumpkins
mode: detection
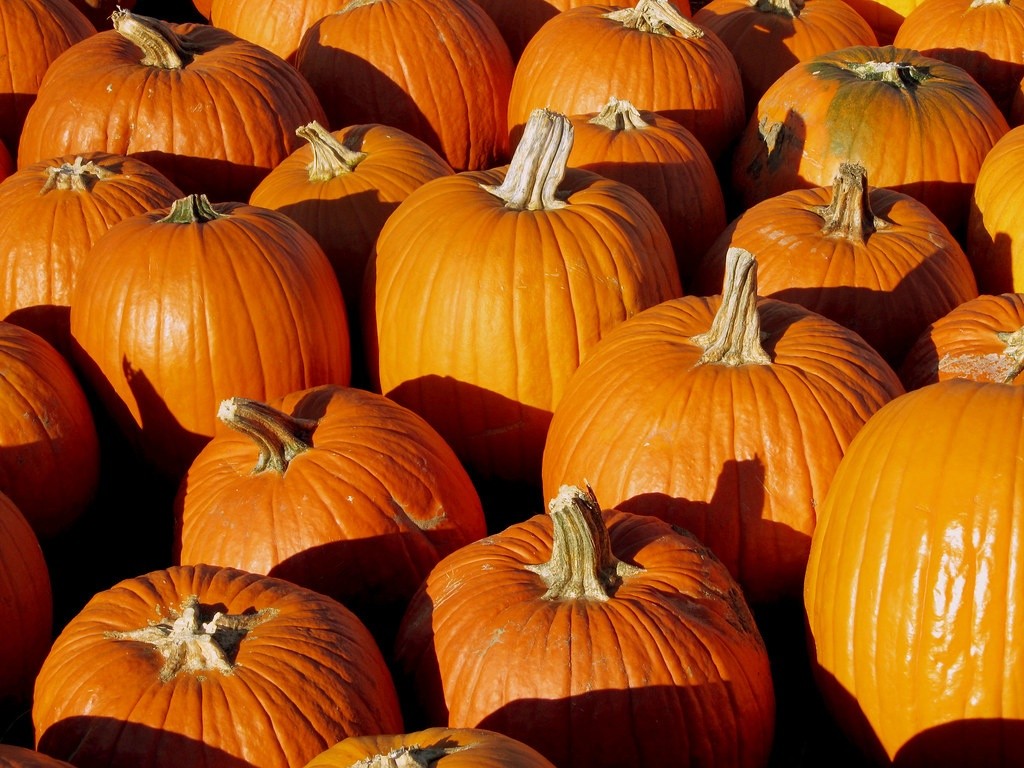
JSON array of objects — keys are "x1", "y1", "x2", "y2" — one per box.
[{"x1": 0, "y1": 0, "x2": 1024, "y2": 768}]
